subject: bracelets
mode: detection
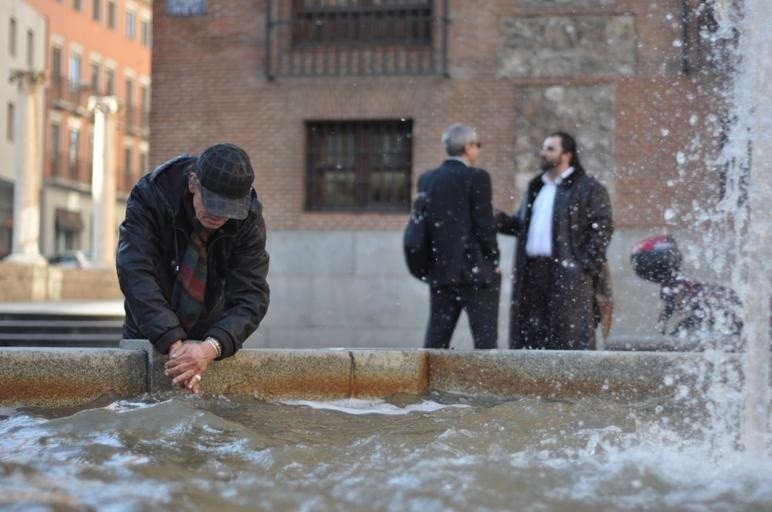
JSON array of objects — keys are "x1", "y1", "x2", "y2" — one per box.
[{"x1": 207, "y1": 336, "x2": 222, "y2": 358}]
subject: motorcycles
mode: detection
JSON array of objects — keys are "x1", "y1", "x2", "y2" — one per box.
[{"x1": 630, "y1": 234, "x2": 745, "y2": 336}]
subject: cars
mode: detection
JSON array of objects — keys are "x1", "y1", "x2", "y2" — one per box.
[{"x1": 48, "y1": 250, "x2": 91, "y2": 269}]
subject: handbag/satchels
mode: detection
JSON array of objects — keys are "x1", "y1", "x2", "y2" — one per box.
[{"x1": 404, "y1": 219, "x2": 429, "y2": 276}]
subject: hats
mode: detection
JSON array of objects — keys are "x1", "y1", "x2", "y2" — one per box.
[{"x1": 196, "y1": 143, "x2": 253, "y2": 221}]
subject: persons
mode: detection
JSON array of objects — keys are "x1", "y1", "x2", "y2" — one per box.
[
  {"x1": 494, "y1": 131, "x2": 614, "y2": 350},
  {"x1": 115, "y1": 144, "x2": 270, "y2": 398},
  {"x1": 414, "y1": 122, "x2": 502, "y2": 349}
]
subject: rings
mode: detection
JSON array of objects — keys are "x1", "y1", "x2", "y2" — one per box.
[{"x1": 196, "y1": 374, "x2": 201, "y2": 382}]
